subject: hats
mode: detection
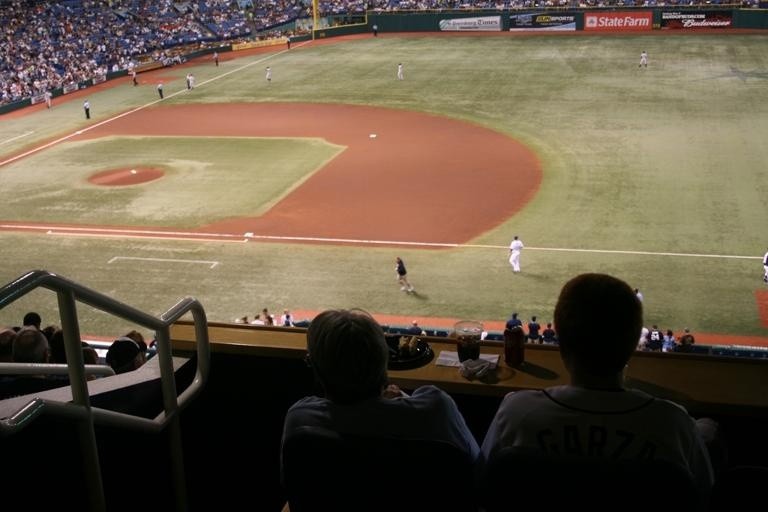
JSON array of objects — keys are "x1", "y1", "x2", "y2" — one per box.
[{"x1": 105, "y1": 336, "x2": 148, "y2": 366}]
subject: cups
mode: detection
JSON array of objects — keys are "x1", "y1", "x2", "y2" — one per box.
[
  {"x1": 453, "y1": 319, "x2": 483, "y2": 363},
  {"x1": 502, "y1": 327, "x2": 525, "y2": 366}
]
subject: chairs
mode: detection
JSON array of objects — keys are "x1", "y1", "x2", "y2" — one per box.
[
  {"x1": 509, "y1": 444, "x2": 698, "y2": 511},
  {"x1": 259, "y1": 439, "x2": 490, "y2": 510}
]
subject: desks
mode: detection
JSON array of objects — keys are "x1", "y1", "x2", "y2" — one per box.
[{"x1": 169, "y1": 322, "x2": 768, "y2": 408}]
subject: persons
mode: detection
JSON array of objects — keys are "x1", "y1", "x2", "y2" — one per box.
[
  {"x1": 274, "y1": 307, "x2": 482, "y2": 512},
  {"x1": 234, "y1": 306, "x2": 295, "y2": 327},
  {"x1": 506, "y1": 235, "x2": 526, "y2": 274},
  {"x1": 761, "y1": 247, "x2": 768, "y2": 283},
  {"x1": 409, "y1": 320, "x2": 423, "y2": 335},
  {"x1": 372, "y1": 23, "x2": 378, "y2": 37},
  {"x1": 392, "y1": 256, "x2": 415, "y2": 292},
  {"x1": 633, "y1": 287, "x2": 643, "y2": 300},
  {"x1": 0, "y1": 302, "x2": 160, "y2": 400},
  {"x1": 315, "y1": 0, "x2": 767, "y2": 30},
  {"x1": 639, "y1": 51, "x2": 648, "y2": 68},
  {"x1": 459, "y1": 272, "x2": 721, "y2": 512},
  {"x1": 397, "y1": 62, "x2": 405, "y2": 81},
  {"x1": 500, "y1": 312, "x2": 697, "y2": 353},
  {"x1": 83, "y1": 99, "x2": 90, "y2": 119},
  {"x1": 1, "y1": 1, "x2": 313, "y2": 115}
]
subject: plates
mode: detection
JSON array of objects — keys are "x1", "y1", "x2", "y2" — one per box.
[{"x1": 381, "y1": 336, "x2": 434, "y2": 369}]
